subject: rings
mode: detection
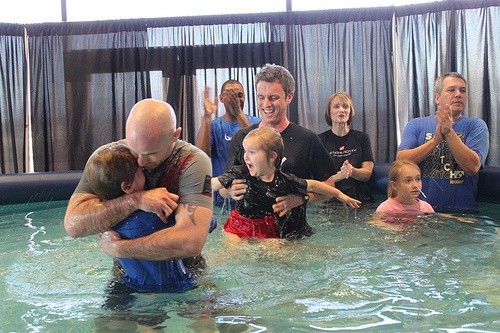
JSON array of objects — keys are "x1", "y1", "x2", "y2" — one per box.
[{"x1": 285, "y1": 204, "x2": 287, "y2": 208}]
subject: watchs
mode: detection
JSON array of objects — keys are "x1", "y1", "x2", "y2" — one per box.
[{"x1": 302, "y1": 194, "x2": 310, "y2": 204}]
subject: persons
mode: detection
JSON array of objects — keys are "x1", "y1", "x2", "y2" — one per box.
[
  {"x1": 211, "y1": 127, "x2": 361, "y2": 251},
  {"x1": 195, "y1": 79, "x2": 262, "y2": 210},
  {"x1": 396, "y1": 72, "x2": 489, "y2": 214},
  {"x1": 317, "y1": 91, "x2": 375, "y2": 213},
  {"x1": 85, "y1": 144, "x2": 218, "y2": 333},
  {"x1": 219, "y1": 63, "x2": 338, "y2": 251},
  {"x1": 367, "y1": 158, "x2": 477, "y2": 231},
  {"x1": 64, "y1": 98, "x2": 255, "y2": 333}
]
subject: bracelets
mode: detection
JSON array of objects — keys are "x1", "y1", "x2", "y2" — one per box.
[{"x1": 443, "y1": 127, "x2": 453, "y2": 137}]
